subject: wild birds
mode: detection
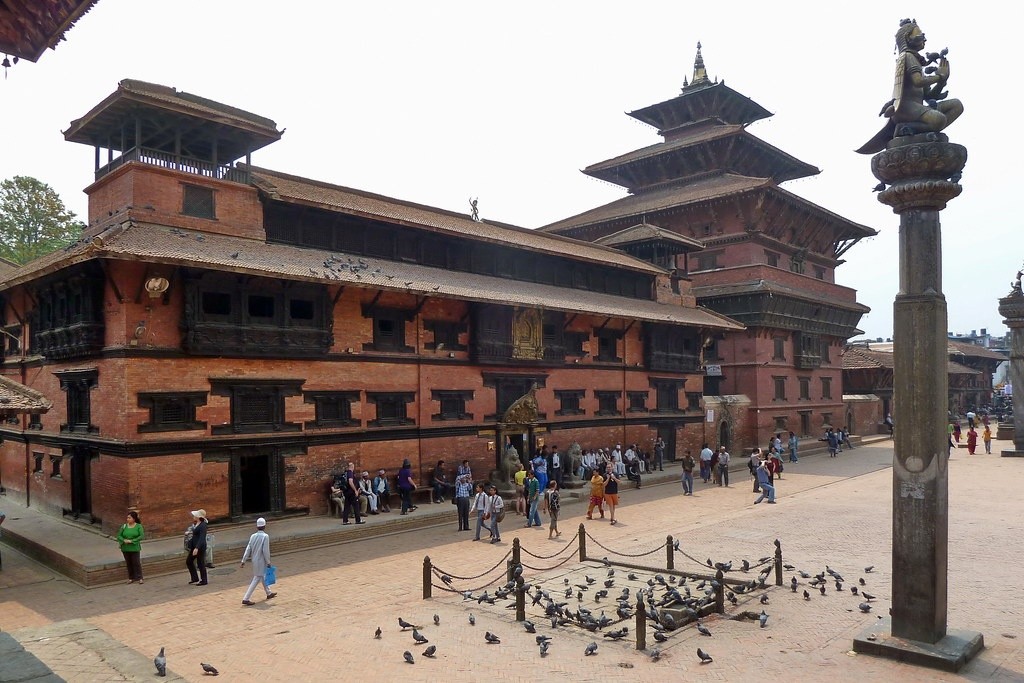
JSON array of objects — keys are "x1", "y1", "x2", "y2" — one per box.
[
  {"x1": 422, "y1": 644, "x2": 437, "y2": 658},
  {"x1": 478, "y1": 539, "x2": 892, "y2": 664},
  {"x1": 398, "y1": 617, "x2": 419, "y2": 631},
  {"x1": 412, "y1": 628, "x2": 428, "y2": 644},
  {"x1": 469, "y1": 613, "x2": 476, "y2": 624},
  {"x1": 199, "y1": 662, "x2": 219, "y2": 676},
  {"x1": 403, "y1": 651, "x2": 415, "y2": 664},
  {"x1": 433, "y1": 614, "x2": 440, "y2": 626},
  {"x1": 154, "y1": 647, "x2": 166, "y2": 677},
  {"x1": 375, "y1": 626, "x2": 382, "y2": 639},
  {"x1": 485, "y1": 631, "x2": 501, "y2": 644},
  {"x1": 463, "y1": 588, "x2": 473, "y2": 600}
]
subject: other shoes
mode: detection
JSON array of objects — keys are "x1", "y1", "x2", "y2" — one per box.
[
  {"x1": 189, "y1": 580, "x2": 198, "y2": 584},
  {"x1": 753, "y1": 447, "x2": 855, "y2": 505},
  {"x1": 458, "y1": 512, "x2": 619, "y2": 544},
  {"x1": 646, "y1": 466, "x2": 728, "y2": 496},
  {"x1": 139, "y1": 579, "x2": 143, "y2": 583},
  {"x1": 337, "y1": 496, "x2": 444, "y2": 525},
  {"x1": 267, "y1": 592, "x2": 278, "y2": 599},
  {"x1": 206, "y1": 562, "x2": 215, "y2": 568},
  {"x1": 127, "y1": 579, "x2": 134, "y2": 584},
  {"x1": 242, "y1": 600, "x2": 255, "y2": 605},
  {"x1": 196, "y1": 581, "x2": 205, "y2": 586}
]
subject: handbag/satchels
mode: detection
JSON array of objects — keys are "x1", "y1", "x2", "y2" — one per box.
[
  {"x1": 700, "y1": 458, "x2": 705, "y2": 469},
  {"x1": 183, "y1": 526, "x2": 193, "y2": 550},
  {"x1": 452, "y1": 497, "x2": 458, "y2": 505},
  {"x1": 118, "y1": 524, "x2": 126, "y2": 549},
  {"x1": 496, "y1": 510, "x2": 506, "y2": 522},
  {"x1": 748, "y1": 459, "x2": 752, "y2": 472},
  {"x1": 482, "y1": 512, "x2": 490, "y2": 521},
  {"x1": 265, "y1": 566, "x2": 276, "y2": 587}
]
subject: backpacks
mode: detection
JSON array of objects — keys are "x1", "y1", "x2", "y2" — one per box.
[{"x1": 338, "y1": 471, "x2": 348, "y2": 491}]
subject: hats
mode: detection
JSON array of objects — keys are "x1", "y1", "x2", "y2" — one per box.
[
  {"x1": 362, "y1": 472, "x2": 369, "y2": 477},
  {"x1": 191, "y1": 510, "x2": 207, "y2": 519},
  {"x1": 379, "y1": 470, "x2": 385, "y2": 475},
  {"x1": 257, "y1": 518, "x2": 266, "y2": 527},
  {"x1": 616, "y1": 445, "x2": 620, "y2": 449},
  {"x1": 605, "y1": 447, "x2": 609, "y2": 451},
  {"x1": 598, "y1": 449, "x2": 604, "y2": 453}
]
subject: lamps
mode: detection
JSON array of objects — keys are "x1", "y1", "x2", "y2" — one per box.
[
  {"x1": 347, "y1": 347, "x2": 354, "y2": 353},
  {"x1": 143, "y1": 273, "x2": 170, "y2": 298},
  {"x1": 574, "y1": 359, "x2": 580, "y2": 363},
  {"x1": 636, "y1": 362, "x2": 640, "y2": 366},
  {"x1": 135, "y1": 327, "x2": 147, "y2": 337},
  {"x1": 449, "y1": 352, "x2": 456, "y2": 358}
]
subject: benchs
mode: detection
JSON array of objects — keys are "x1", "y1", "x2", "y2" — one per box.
[{"x1": 331, "y1": 481, "x2": 485, "y2": 520}]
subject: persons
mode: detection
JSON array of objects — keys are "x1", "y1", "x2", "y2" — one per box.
[
  {"x1": 328, "y1": 425, "x2": 666, "y2": 549},
  {"x1": 680, "y1": 445, "x2": 731, "y2": 496},
  {"x1": 856, "y1": 20, "x2": 963, "y2": 155},
  {"x1": 240, "y1": 517, "x2": 277, "y2": 605},
  {"x1": 184, "y1": 509, "x2": 215, "y2": 586},
  {"x1": 747, "y1": 432, "x2": 799, "y2": 505},
  {"x1": 948, "y1": 393, "x2": 1013, "y2": 455},
  {"x1": 825, "y1": 426, "x2": 854, "y2": 458},
  {"x1": 1008, "y1": 271, "x2": 1024, "y2": 297},
  {"x1": 886, "y1": 412, "x2": 895, "y2": 438},
  {"x1": 117, "y1": 512, "x2": 144, "y2": 585}
]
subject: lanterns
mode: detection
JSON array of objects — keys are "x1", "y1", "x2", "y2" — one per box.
[{"x1": 145, "y1": 277, "x2": 169, "y2": 298}]
